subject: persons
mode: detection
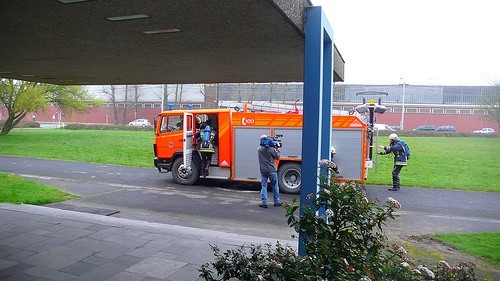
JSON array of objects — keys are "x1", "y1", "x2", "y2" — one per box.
[
  {"x1": 177, "y1": 117, "x2": 183, "y2": 130},
  {"x1": 32, "y1": 114, "x2": 36, "y2": 122},
  {"x1": 377, "y1": 133, "x2": 408, "y2": 191},
  {"x1": 193, "y1": 115, "x2": 215, "y2": 177},
  {"x1": 52, "y1": 113, "x2": 56, "y2": 122},
  {"x1": 258, "y1": 135, "x2": 283, "y2": 208}
]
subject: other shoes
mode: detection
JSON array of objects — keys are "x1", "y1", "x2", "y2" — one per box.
[
  {"x1": 388, "y1": 187, "x2": 398, "y2": 191},
  {"x1": 206, "y1": 168, "x2": 209, "y2": 176},
  {"x1": 398, "y1": 187, "x2": 400, "y2": 189},
  {"x1": 274, "y1": 202, "x2": 283, "y2": 207},
  {"x1": 259, "y1": 203, "x2": 268, "y2": 208}
]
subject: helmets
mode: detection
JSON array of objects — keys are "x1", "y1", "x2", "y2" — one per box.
[{"x1": 389, "y1": 133, "x2": 398, "y2": 140}]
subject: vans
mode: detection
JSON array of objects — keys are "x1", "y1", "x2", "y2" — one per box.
[{"x1": 372, "y1": 124, "x2": 397, "y2": 132}]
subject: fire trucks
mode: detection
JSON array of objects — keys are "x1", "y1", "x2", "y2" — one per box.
[{"x1": 152, "y1": 100, "x2": 373, "y2": 194}]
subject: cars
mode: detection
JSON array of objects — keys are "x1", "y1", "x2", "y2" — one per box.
[
  {"x1": 128, "y1": 119, "x2": 151, "y2": 128},
  {"x1": 434, "y1": 125, "x2": 456, "y2": 131},
  {"x1": 413, "y1": 125, "x2": 436, "y2": 131},
  {"x1": 390, "y1": 125, "x2": 400, "y2": 130}
]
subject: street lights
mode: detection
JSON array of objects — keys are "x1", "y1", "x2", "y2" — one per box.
[{"x1": 400, "y1": 77, "x2": 406, "y2": 131}]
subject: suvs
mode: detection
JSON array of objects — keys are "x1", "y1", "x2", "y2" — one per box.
[{"x1": 472, "y1": 128, "x2": 495, "y2": 135}]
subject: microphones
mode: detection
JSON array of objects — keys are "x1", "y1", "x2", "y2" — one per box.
[{"x1": 276, "y1": 135, "x2": 284, "y2": 137}]
